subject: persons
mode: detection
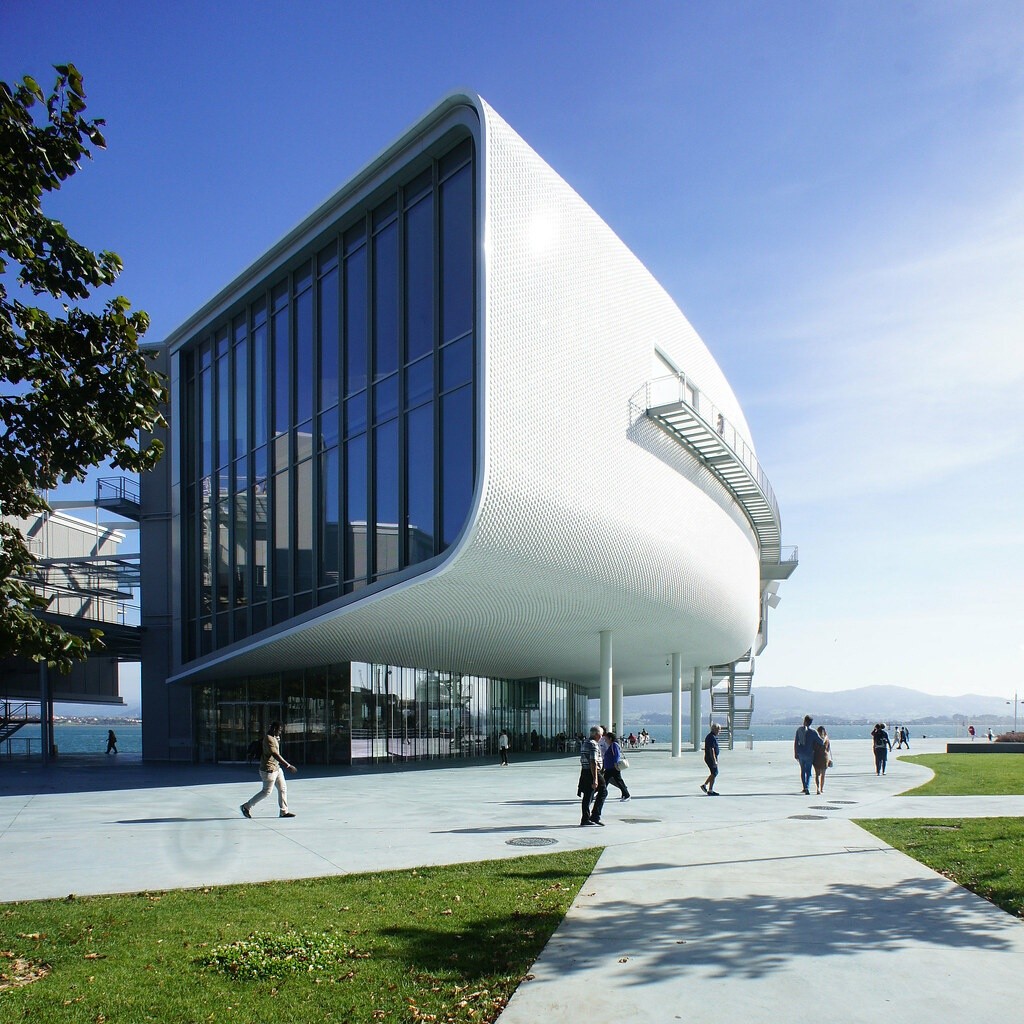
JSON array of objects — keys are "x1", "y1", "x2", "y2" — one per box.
[
  {"x1": 521, "y1": 729, "x2": 560, "y2": 752},
  {"x1": 794, "y1": 715, "x2": 830, "y2": 795},
  {"x1": 593, "y1": 726, "x2": 631, "y2": 802},
  {"x1": 628, "y1": 728, "x2": 648, "y2": 747},
  {"x1": 105, "y1": 730, "x2": 118, "y2": 754},
  {"x1": 240, "y1": 722, "x2": 297, "y2": 818},
  {"x1": 557, "y1": 731, "x2": 583, "y2": 753},
  {"x1": 580, "y1": 725, "x2": 608, "y2": 826},
  {"x1": 701, "y1": 723, "x2": 721, "y2": 795},
  {"x1": 871, "y1": 723, "x2": 992, "y2": 776},
  {"x1": 499, "y1": 729, "x2": 509, "y2": 766}
]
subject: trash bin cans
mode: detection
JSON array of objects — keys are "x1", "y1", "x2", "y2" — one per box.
[{"x1": 53, "y1": 745, "x2": 58, "y2": 756}]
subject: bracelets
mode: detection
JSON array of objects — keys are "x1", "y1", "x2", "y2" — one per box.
[{"x1": 286, "y1": 764, "x2": 290, "y2": 768}]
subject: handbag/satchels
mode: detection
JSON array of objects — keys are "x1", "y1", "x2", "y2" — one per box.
[
  {"x1": 612, "y1": 742, "x2": 629, "y2": 771},
  {"x1": 828, "y1": 759, "x2": 834, "y2": 766}
]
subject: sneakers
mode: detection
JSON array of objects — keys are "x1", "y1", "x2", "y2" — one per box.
[
  {"x1": 589, "y1": 817, "x2": 604, "y2": 826},
  {"x1": 580, "y1": 822, "x2": 595, "y2": 826},
  {"x1": 620, "y1": 795, "x2": 631, "y2": 802},
  {"x1": 802, "y1": 789, "x2": 806, "y2": 792},
  {"x1": 700, "y1": 785, "x2": 707, "y2": 793},
  {"x1": 280, "y1": 812, "x2": 296, "y2": 817},
  {"x1": 240, "y1": 805, "x2": 251, "y2": 818},
  {"x1": 805, "y1": 790, "x2": 810, "y2": 795},
  {"x1": 707, "y1": 791, "x2": 719, "y2": 796}
]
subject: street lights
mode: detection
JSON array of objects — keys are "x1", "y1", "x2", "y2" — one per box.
[{"x1": 1006, "y1": 693, "x2": 1024, "y2": 734}]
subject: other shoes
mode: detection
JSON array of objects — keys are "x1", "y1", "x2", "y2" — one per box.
[
  {"x1": 907, "y1": 747, "x2": 909, "y2": 749},
  {"x1": 897, "y1": 747, "x2": 901, "y2": 749},
  {"x1": 821, "y1": 789, "x2": 824, "y2": 792},
  {"x1": 502, "y1": 762, "x2": 508, "y2": 767},
  {"x1": 877, "y1": 772, "x2": 886, "y2": 776},
  {"x1": 817, "y1": 790, "x2": 820, "y2": 794}
]
